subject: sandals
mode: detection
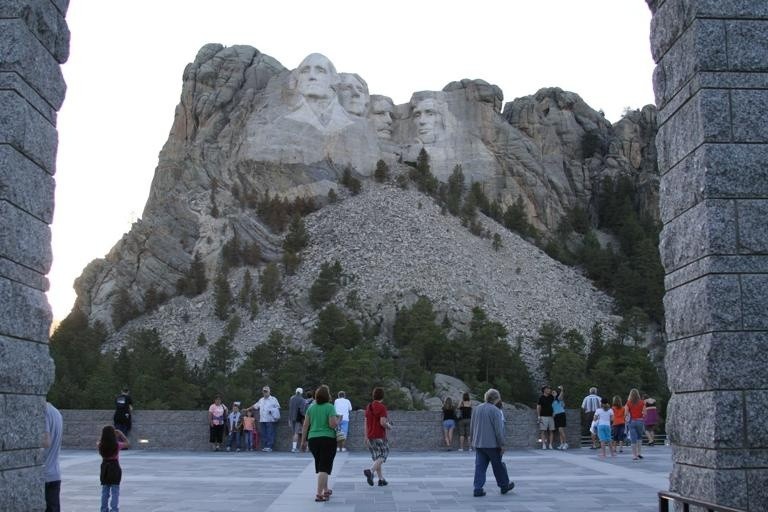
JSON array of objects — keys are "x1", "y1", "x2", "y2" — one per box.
[
  {"x1": 313, "y1": 494, "x2": 329, "y2": 502},
  {"x1": 322, "y1": 488, "x2": 332, "y2": 498}
]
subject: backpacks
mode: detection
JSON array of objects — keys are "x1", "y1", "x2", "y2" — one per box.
[
  {"x1": 263, "y1": 386, "x2": 270, "y2": 392},
  {"x1": 295, "y1": 387, "x2": 303, "y2": 394}
]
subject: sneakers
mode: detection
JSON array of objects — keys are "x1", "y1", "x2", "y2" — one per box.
[
  {"x1": 376, "y1": 478, "x2": 388, "y2": 486},
  {"x1": 336, "y1": 447, "x2": 347, "y2": 452},
  {"x1": 364, "y1": 468, "x2": 375, "y2": 487},
  {"x1": 446, "y1": 446, "x2": 473, "y2": 453},
  {"x1": 473, "y1": 490, "x2": 488, "y2": 497},
  {"x1": 211, "y1": 444, "x2": 273, "y2": 453},
  {"x1": 591, "y1": 440, "x2": 671, "y2": 461},
  {"x1": 287, "y1": 445, "x2": 309, "y2": 453},
  {"x1": 541, "y1": 442, "x2": 569, "y2": 451},
  {"x1": 500, "y1": 481, "x2": 515, "y2": 495}
]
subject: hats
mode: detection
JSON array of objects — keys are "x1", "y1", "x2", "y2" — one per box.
[{"x1": 542, "y1": 385, "x2": 552, "y2": 393}]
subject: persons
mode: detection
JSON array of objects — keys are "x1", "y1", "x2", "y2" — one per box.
[
  {"x1": 113, "y1": 387, "x2": 133, "y2": 451},
  {"x1": 537, "y1": 384, "x2": 658, "y2": 461},
  {"x1": 207, "y1": 385, "x2": 353, "y2": 453},
  {"x1": 469, "y1": 388, "x2": 515, "y2": 497},
  {"x1": 363, "y1": 387, "x2": 394, "y2": 487},
  {"x1": 96, "y1": 425, "x2": 130, "y2": 512},
  {"x1": 441, "y1": 391, "x2": 505, "y2": 451},
  {"x1": 42, "y1": 400, "x2": 63, "y2": 512},
  {"x1": 301, "y1": 385, "x2": 343, "y2": 502}
]
